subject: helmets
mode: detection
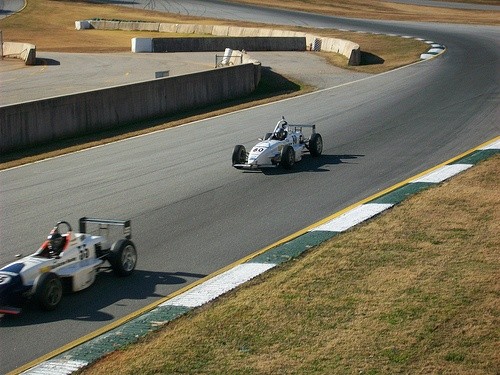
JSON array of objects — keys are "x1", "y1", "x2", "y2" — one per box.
[
  {"x1": 275, "y1": 128, "x2": 285, "y2": 138},
  {"x1": 47, "y1": 232, "x2": 63, "y2": 251}
]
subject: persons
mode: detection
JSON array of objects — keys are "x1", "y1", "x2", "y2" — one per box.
[
  {"x1": 42, "y1": 233, "x2": 65, "y2": 258},
  {"x1": 274, "y1": 127, "x2": 284, "y2": 140}
]
